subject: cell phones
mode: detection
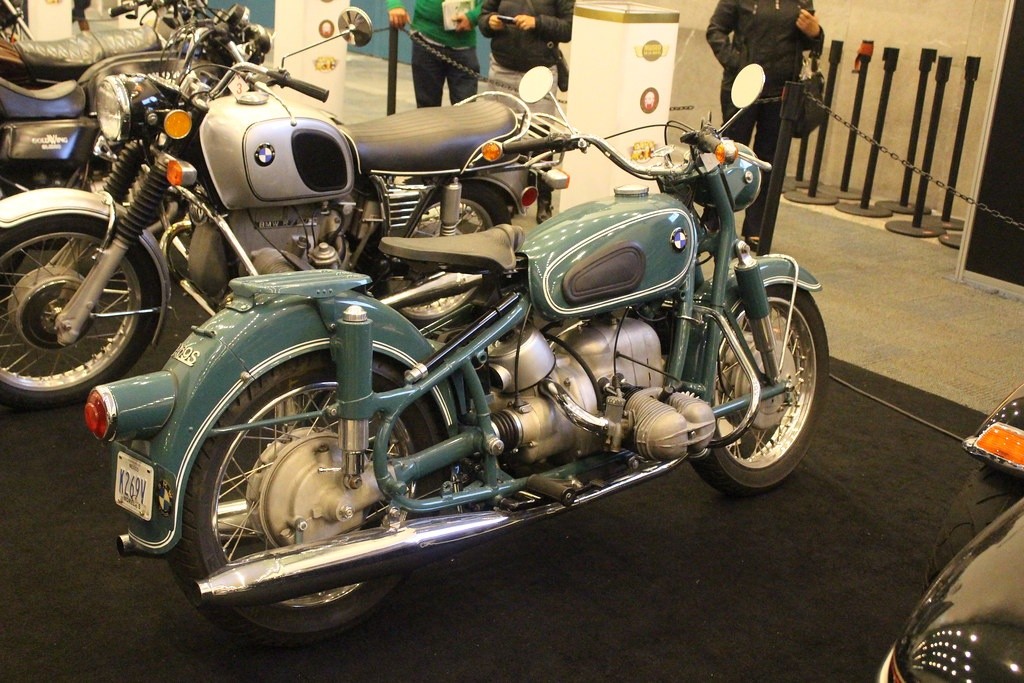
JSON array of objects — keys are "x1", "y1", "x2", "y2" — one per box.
[
  {"x1": 497, "y1": 15, "x2": 515, "y2": 24},
  {"x1": 806, "y1": 9, "x2": 816, "y2": 16}
]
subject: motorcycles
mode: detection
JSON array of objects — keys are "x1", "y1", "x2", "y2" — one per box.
[
  {"x1": 0, "y1": 0, "x2": 832, "y2": 650},
  {"x1": 873, "y1": 384, "x2": 1024, "y2": 683}
]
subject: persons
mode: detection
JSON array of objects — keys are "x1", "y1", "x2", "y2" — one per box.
[
  {"x1": 387, "y1": 0, "x2": 483, "y2": 109},
  {"x1": 706, "y1": 0, "x2": 825, "y2": 257},
  {"x1": 71, "y1": 0, "x2": 92, "y2": 31},
  {"x1": 479, "y1": 0, "x2": 575, "y2": 224}
]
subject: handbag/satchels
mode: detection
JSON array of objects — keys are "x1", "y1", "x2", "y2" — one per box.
[
  {"x1": 790, "y1": 24, "x2": 828, "y2": 138},
  {"x1": 556, "y1": 48, "x2": 568, "y2": 92}
]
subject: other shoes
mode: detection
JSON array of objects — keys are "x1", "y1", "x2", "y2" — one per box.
[{"x1": 745, "y1": 236, "x2": 759, "y2": 252}]
